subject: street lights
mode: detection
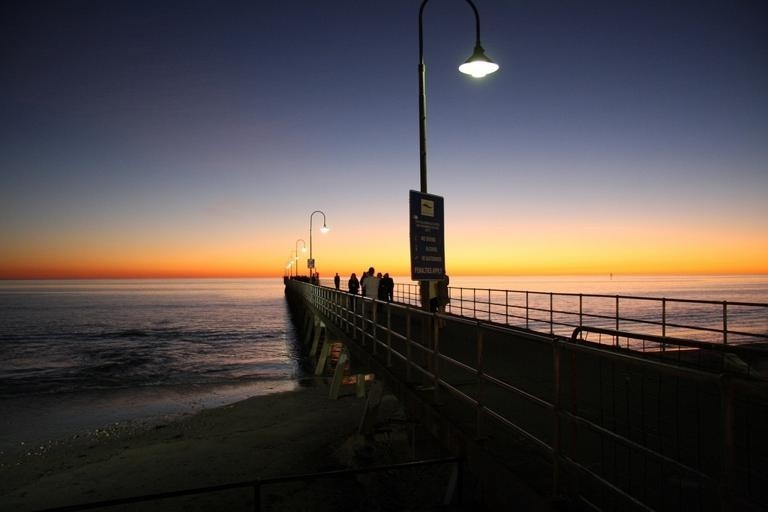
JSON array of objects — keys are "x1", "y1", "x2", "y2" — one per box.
[
  {"x1": 416, "y1": 1, "x2": 501, "y2": 311},
  {"x1": 285, "y1": 238, "x2": 308, "y2": 277},
  {"x1": 309, "y1": 208, "x2": 330, "y2": 282}
]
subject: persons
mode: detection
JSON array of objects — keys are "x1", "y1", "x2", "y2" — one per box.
[
  {"x1": 419, "y1": 275, "x2": 449, "y2": 314},
  {"x1": 348, "y1": 267, "x2": 394, "y2": 302},
  {"x1": 311, "y1": 272, "x2": 319, "y2": 285},
  {"x1": 334, "y1": 273, "x2": 340, "y2": 291}
]
